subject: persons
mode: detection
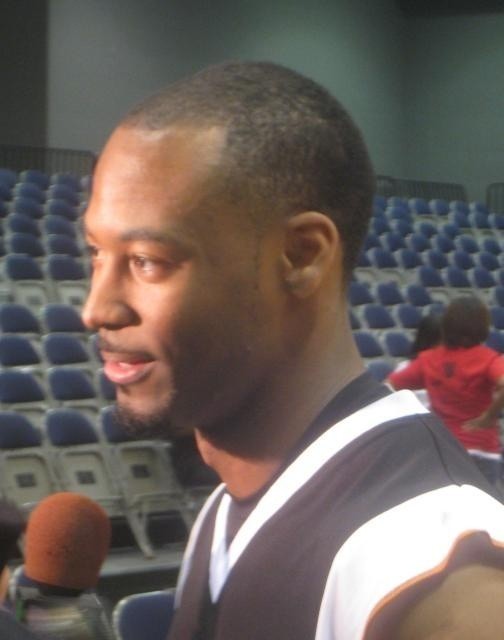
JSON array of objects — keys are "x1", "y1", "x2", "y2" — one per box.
[
  {"x1": 0, "y1": 491, "x2": 112, "y2": 640},
  {"x1": 80, "y1": 60, "x2": 504, "y2": 640},
  {"x1": 383, "y1": 296, "x2": 504, "y2": 454}
]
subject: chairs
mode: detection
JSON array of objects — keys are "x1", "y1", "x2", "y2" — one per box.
[
  {"x1": 111, "y1": 589, "x2": 181, "y2": 640},
  {"x1": 0, "y1": 166, "x2": 119, "y2": 409},
  {"x1": 100, "y1": 402, "x2": 196, "y2": 556},
  {"x1": 42, "y1": 408, "x2": 156, "y2": 558},
  {"x1": 347, "y1": 197, "x2": 503, "y2": 381},
  {"x1": 1, "y1": 411, "x2": 63, "y2": 562}
]
